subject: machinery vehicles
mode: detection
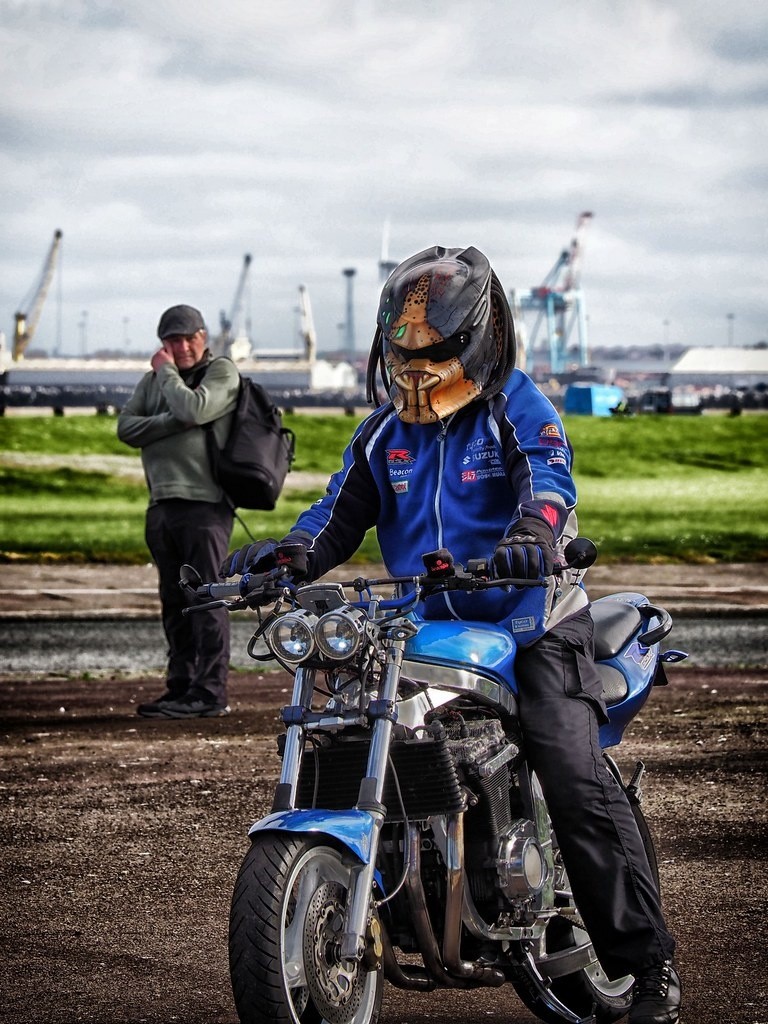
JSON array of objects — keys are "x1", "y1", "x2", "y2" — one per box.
[
  {"x1": 212, "y1": 254, "x2": 255, "y2": 362},
  {"x1": 9, "y1": 227, "x2": 65, "y2": 361},
  {"x1": 294, "y1": 284, "x2": 318, "y2": 365}
]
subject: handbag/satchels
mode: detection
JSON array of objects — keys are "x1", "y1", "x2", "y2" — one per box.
[{"x1": 193, "y1": 356, "x2": 297, "y2": 510}]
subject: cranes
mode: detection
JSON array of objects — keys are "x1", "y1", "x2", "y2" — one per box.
[{"x1": 508, "y1": 207, "x2": 597, "y2": 377}]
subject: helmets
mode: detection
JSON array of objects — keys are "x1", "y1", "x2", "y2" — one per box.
[{"x1": 366, "y1": 247, "x2": 516, "y2": 425}]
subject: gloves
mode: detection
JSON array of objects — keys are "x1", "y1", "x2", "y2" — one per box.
[
  {"x1": 488, "y1": 518, "x2": 555, "y2": 593},
  {"x1": 217, "y1": 530, "x2": 330, "y2": 584}
]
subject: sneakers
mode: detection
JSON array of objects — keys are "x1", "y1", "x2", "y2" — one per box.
[
  {"x1": 137, "y1": 692, "x2": 184, "y2": 716},
  {"x1": 157, "y1": 688, "x2": 231, "y2": 718},
  {"x1": 627, "y1": 958, "x2": 682, "y2": 1024}
]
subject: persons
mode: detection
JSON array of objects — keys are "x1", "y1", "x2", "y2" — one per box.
[
  {"x1": 221, "y1": 248, "x2": 683, "y2": 1023},
  {"x1": 117, "y1": 306, "x2": 241, "y2": 719}
]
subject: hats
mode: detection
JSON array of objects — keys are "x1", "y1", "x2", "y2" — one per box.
[{"x1": 157, "y1": 305, "x2": 204, "y2": 339}]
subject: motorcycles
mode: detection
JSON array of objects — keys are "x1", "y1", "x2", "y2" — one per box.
[{"x1": 175, "y1": 534, "x2": 692, "y2": 1024}]
